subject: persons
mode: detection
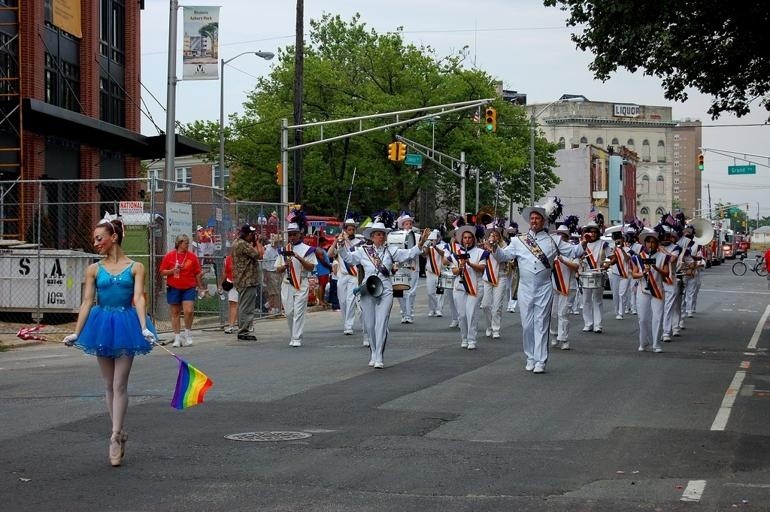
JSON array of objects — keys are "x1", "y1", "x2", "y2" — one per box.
[
  {"x1": 158, "y1": 232, "x2": 204, "y2": 348},
  {"x1": 336, "y1": 221, "x2": 432, "y2": 369},
  {"x1": 62, "y1": 214, "x2": 159, "y2": 466},
  {"x1": 488, "y1": 204, "x2": 592, "y2": 373}
]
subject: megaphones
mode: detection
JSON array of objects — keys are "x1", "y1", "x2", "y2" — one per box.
[{"x1": 353, "y1": 275, "x2": 384, "y2": 298}]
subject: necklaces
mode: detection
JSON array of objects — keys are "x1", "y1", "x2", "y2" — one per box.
[{"x1": 175, "y1": 250, "x2": 187, "y2": 268}]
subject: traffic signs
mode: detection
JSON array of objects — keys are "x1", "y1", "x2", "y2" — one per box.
[
  {"x1": 308, "y1": 220, "x2": 327, "y2": 226},
  {"x1": 404, "y1": 154, "x2": 423, "y2": 165},
  {"x1": 728, "y1": 165, "x2": 756, "y2": 174}
]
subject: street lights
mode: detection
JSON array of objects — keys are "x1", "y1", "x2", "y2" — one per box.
[
  {"x1": 697, "y1": 198, "x2": 711, "y2": 218},
  {"x1": 530, "y1": 97, "x2": 584, "y2": 207},
  {"x1": 219, "y1": 50, "x2": 275, "y2": 322}
]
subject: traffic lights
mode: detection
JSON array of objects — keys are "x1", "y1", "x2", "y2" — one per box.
[
  {"x1": 469, "y1": 215, "x2": 477, "y2": 223},
  {"x1": 486, "y1": 109, "x2": 496, "y2": 133},
  {"x1": 698, "y1": 154, "x2": 704, "y2": 171},
  {"x1": 398, "y1": 143, "x2": 407, "y2": 162},
  {"x1": 388, "y1": 143, "x2": 394, "y2": 161}
]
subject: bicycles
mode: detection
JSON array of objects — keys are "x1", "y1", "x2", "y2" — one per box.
[{"x1": 732, "y1": 252, "x2": 769, "y2": 277}]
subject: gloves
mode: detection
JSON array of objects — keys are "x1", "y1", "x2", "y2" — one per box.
[
  {"x1": 143, "y1": 328, "x2": 156, "y2": 342},
  {"x1": 64, "y1": 334, "x2": 77, "y2": 346}
]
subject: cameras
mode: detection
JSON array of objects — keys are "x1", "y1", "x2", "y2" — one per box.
[{"x1": 252, "y1": 232, "x2": 270, "y2": 247}]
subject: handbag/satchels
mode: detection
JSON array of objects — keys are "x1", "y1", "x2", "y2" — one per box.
[{"x1": 221, "y1": 277, "x2": 234, "y2": 292}]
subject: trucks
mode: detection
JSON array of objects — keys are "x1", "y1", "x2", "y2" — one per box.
[
  {"x1": 224, "y1": 215, "x2": 344, "y2": 263},
  {"x1": 600, "y1": 218, "x2": 751, "y2": 297}
]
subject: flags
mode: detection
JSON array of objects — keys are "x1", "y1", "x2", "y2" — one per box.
[{"x1": 169, "y1": 356, "x2": 214, "y2": 408}]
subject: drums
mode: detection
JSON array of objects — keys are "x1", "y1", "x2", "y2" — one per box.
[
  {"x1": 389, "y1": 266, "x2": 412, "y2": 290},
  {"x1": 386, "y1": 229, "x2": 417, "y2": 250},
  {"x1": 577, "y1": 270, "x2": 605, "y2": 288},
  {"x1": 436, "y1": 276, "x2": 456, "y2": 289}
]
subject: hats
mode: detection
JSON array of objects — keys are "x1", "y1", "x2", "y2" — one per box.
[
  {"x1": 397, "y1": 214, "x2": 414, "y2": 228},
  {"x1": 241, "y1": 225, "x2": 257, "y2": 233},
  {"x1": 364, "y1": 222, "x2": 392, "y2": 239},
  {"x1": 450, "y1": 206, "x2": 694, "y2": 245},
  {"x1": 286, "y1": 223, "x2": 300, "y2": 232},
  {"x1": 344, "y1": 219, "x2": 357, "y2": 226}
]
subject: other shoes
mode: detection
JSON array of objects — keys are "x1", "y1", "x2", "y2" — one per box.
[
  {"x1": 663, "y1": 336, "x2": 671, "y2": 342},
  {"x1": 461, "y1": 340, "x2": 468, "y2": 347},
  {"x1": 594, "y1": 327, "x2": 602, "y2": 332},
  {"x1": 638, "y1": 346, "x2": 648, "y2": 351},
  {"x1": 436, "y1": 311, "x2": 442, "y2": 317},
  {"x1": 486, "y1": 328, "x2": 492, "y2": 337},
  {"x1": 467, "y1": 343, "x2": 476, "y2": 349},
  {"x1": 574, "y1": 310, "x2": 579, "y2": 315},
  {"x1": 582, "y1": 326, "x2": 593, "y2": 332},
  {"x1": 507, "y1": 308, "x2": 515, "y2": 313},
  {"x1": 492, "y1": 332, "x2": 500, "y2": 339},
  {"x1": 652, "y1": 348, "x2": 662, "y2": 352},
  {"x1": 401, "y1": 317, "x2": 412, "y2": 323},
  {"x1": 560, "y1": 341, "x2": 569, "y2": 350},
  {"x1": 632, "y1": 310, "x2": 637, "y2": 315},
  {"x1": 449, "y1": 320, "x2": 459, "y2": 328},
  {"x1": 238, "y1": 334, "x2": 256, "y2": 341},
  {"x1": 551, "y1": 334, "x2": 559, "y2": 346},
  {"x1": 673, "y1": 332, "x2": 680, "y2": 337},
  {"x1": 344, "y1": 330, "x2": 354, "y2": 335},
  {"x1": 289, "y1": 340, "x2": 301, "y2": 346},
  {"x1": 688, "y1": 312, "x2": 694, "y2": 318},
  {"x1": 616, "y1": 315, "x2": 623, "y2": 320},
  {"x1": 428, "y1": 311, "x2": 436, "y2": 316}
]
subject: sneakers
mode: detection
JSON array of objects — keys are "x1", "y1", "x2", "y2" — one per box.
[
  {"x1": 525, "y1": 364, "x2": 535, "y2": 371},
  {"x1": 367, "y1": 360, "x2": 374, "y2": 367},
  {"x1": 173, "y1": 340, "x2": 183, "y2": 347},
  {"x1": 533, "y1": 365, "x2": 545, "y2": 374},
  {"x1": 373, "y1": 361, "x2": 385, "y2": 369},
  {"x1": 109, "y1": 432, "x2": 127, "y2": 466},
  {"x1": 184, "y1": 337, "x2": 193, "y2": 346}
]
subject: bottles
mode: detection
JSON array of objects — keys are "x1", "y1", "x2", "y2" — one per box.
[
  {"x1": 174, "y1": 260, "x2": 181, "y2": 280},
  {"x1": 217, "y1": 288, "x2": 225, "y2": 301}
]
